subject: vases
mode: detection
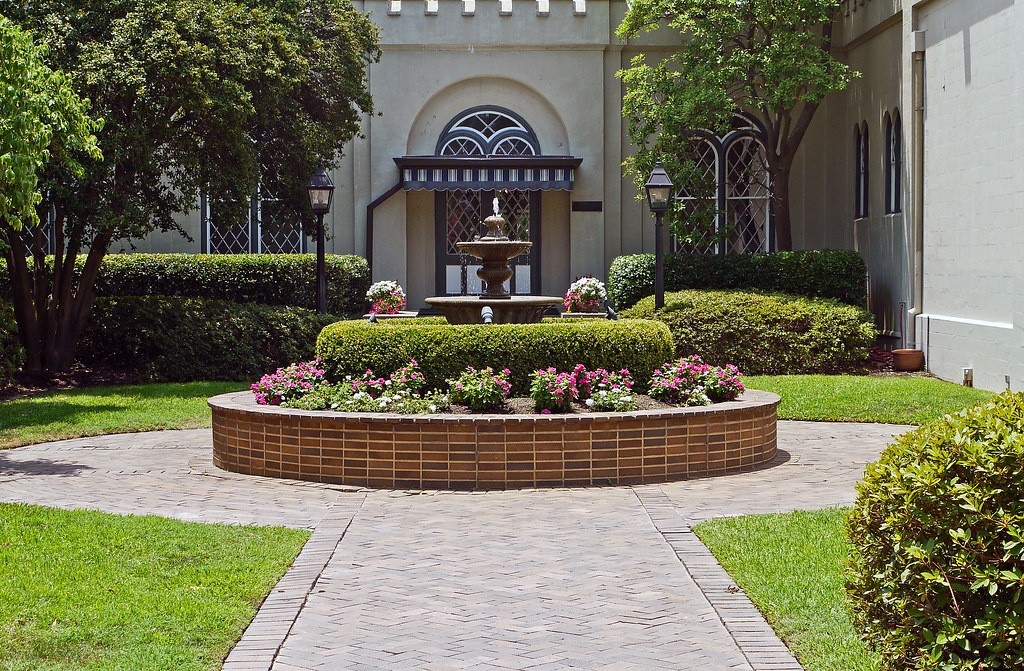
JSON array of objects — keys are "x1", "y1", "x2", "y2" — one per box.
[{"x1": 575, "y1": 305, "x2": 599, "y2": 313}]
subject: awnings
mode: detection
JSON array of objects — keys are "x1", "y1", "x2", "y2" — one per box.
[{"x1": 392, "y1": 154, "x2": 583, "y2": 193}]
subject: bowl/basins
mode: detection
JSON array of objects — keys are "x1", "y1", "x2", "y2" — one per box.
[{"x1": 892, "y1": 349, "x2": 923, "y2": 370}]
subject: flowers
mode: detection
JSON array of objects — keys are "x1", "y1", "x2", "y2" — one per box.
[
  {"x1": 364, "y1": 280, "x2": 407, "y2": 314},
  {"x1": 563, "y1": 277, "x2": 608, "y2": 312}
]
